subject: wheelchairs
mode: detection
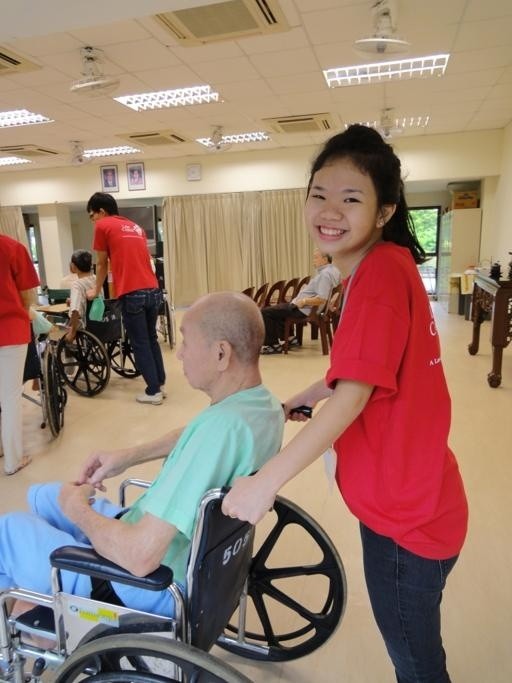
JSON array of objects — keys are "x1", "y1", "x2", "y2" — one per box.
[
  {"x1": 0, "y1": 405, "x2": 350, "y2": 680},
  {"x1": 22, "y1": 288, "x2": 178, "y2": 440}
]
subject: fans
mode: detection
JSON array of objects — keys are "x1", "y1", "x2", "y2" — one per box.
[
  {"x1": 70, "y1": 141, "x2": 91, "y2": 167},
  {"x1": 68, "y1": 48, "x2": 119, "y2": 94},
  {"x1": 378, "y1": 108, "x2": 403, "y2": 139},
  {"x1": 354, "y1": 0, "x2": 409, "y2": 54},
  {"x1": 207, "y1": 126, "x2": 232, "y2": 154}
]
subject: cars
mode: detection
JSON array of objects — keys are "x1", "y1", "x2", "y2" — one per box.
[{"x1": 417, "y1": 265, "x2": 436, "y2": 278}]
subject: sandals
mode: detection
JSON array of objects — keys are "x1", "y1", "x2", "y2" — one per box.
[{"x1": 260, "y1": 336, "x2": 299, "y2": 354}]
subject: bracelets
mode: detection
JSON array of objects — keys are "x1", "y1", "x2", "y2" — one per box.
[{"x1": 302, "y1": 298, "x2": 306, "y2": 304}]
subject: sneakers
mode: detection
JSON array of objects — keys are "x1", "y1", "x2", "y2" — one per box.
[
  {"x1": 5, "y1": 455, "x2": 33, "y2": 474},
  {"x1": 135, "y1": 386, "x2": 166, "y2": 406}
]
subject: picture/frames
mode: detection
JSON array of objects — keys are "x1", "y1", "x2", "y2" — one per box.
[
  {"x1": 100, "y1": 165, "x2": 119, "y2": 193},
  {"x1": 127, "y1": 162, "x2": 145, "y2": 191}
]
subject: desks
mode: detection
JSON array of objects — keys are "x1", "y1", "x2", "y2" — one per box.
[
  {"x1": 36, "y1": 302, "x2": 70, "y2": 317},
  {"x1": 468, "y1": 271, "x2": 512, "y2": 388}
]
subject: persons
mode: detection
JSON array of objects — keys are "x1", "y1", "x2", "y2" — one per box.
[
  {"x1": 63, "y1": 248, "x2": 107, "y2": 345},
  {"x1": 86, "y1": 192, "x2": 169, "y2": 405},
  {"x1": 0, "y1": 232, "x2": 42, "y2": 476},
  {"x1": 131, "y1": 170, "x2": 142, "y2": 186},
  {"x1": 259, "y1": 246, "x2": 342, "y2": 355},
  {"x1": 104, "y1": 168, "x2": 116, "y2": 187},
  {"x1": 0, "y1": 291, "x2": 286, "y2": 650},
  {"x1": 221, "y1": 124, "x2": 469, "y2": 683}
]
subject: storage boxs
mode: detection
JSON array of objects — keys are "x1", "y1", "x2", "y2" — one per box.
[{"x1": 452, "y1": 192, "x2": 477, "y2": 209}]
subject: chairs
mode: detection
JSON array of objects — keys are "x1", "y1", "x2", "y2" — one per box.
[{"x1": 241, "y1": 276, "x2": 342, "y2": 355}]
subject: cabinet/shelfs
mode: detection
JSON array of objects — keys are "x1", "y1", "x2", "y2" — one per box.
[{"x1": 438, "y1": 208, "x2": 481, "y2": 313}]
